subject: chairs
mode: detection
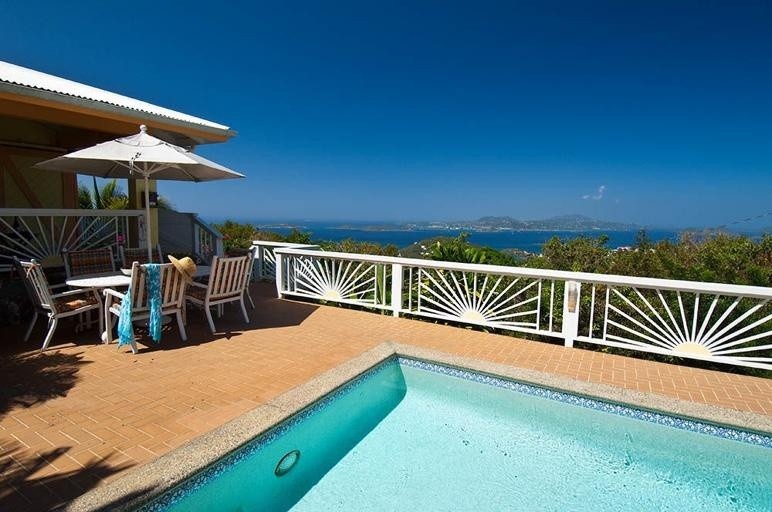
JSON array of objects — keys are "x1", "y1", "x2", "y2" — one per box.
[{"x1": 7, "y1": 243, "x2": 258, "y2": 355}]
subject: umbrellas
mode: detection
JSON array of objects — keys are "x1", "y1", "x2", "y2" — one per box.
[{"x1": 29, "y1": 123, "x2": 251, "y2": 265}]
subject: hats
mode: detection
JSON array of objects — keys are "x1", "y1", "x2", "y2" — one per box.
[{"x1": 166, "y1": 254, "x2": 197, "y2": 284}]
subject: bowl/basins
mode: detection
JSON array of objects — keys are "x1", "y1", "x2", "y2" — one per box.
[{"x1": 120, "y1": 267, "x2": 131, "y2": 276}]
